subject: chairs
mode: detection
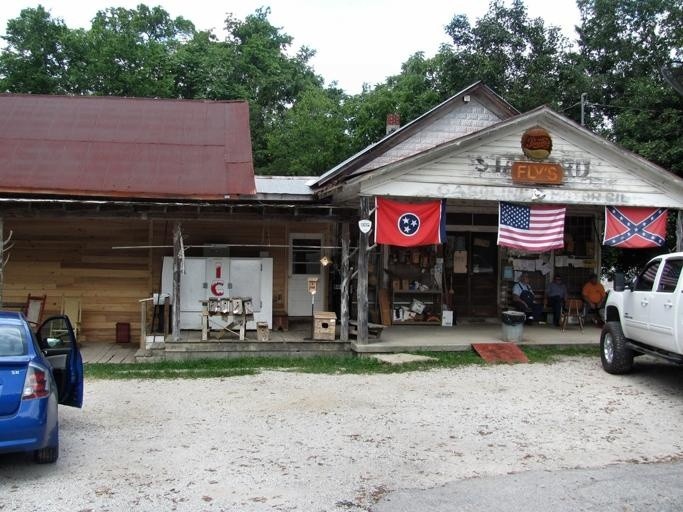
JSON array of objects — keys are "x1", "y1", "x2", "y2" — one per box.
[
  {"x1": 20, "y1": 292, "x2": 83, "y2": 347},
  {"x1": 560, "y1": 298, "x2": 585, "y2": 333}
]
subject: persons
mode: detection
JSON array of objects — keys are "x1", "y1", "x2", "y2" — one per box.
[
  {"x1": 543, "y1": 275, "x2": 569, "y2": 327},
  {"x1": 512, "y1": 272, "x2": 547, "y2": 327},
  {"x1": 582, "y1": 274, "x2": 606, "y2": 327}
]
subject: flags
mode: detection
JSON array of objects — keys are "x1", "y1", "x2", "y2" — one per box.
[
  {"x1": 372, "y1": 196, "x2": 448, "y2": 247},
  {"x1": 641, "y1": 262, "x2": 680, "y2": 288},
  {"x1": 600, "y1": 206, "x2": 669, "y2": 249},
  {"x1": 493, "y1": 202, "x2": 566, "y2": 251}
]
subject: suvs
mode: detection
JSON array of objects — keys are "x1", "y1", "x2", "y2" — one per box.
[{"x1": 600, "y1": 251, "x2": 683, "y2": 375}]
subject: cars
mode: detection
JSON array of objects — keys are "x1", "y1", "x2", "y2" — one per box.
[{"x1": 0, "y1": 311, "x2": 83, "y2": 463}]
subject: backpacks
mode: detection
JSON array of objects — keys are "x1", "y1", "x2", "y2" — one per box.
[{"x1": 516, "y1": 282, "x2": 534, "y2": 308}]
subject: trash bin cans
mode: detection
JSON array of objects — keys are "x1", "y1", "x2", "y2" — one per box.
[
  {"x1": 156, "y1": 305, "x2": 172, "y2": 334},
  {"x1": 501, "y1": 311, "x2": 526, "y2": 342}
]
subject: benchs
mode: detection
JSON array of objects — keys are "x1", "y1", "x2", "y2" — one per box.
[{"x1": 507, "y1": 287, "x2": 582, "y2": 321}]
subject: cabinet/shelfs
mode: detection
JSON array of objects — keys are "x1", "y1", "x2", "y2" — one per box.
[{"x1": 392, "y1": 290, "x2": 443, "y2": 325}]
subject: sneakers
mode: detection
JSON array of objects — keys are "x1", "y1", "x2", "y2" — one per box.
[{"x1": 526, "y1": 314, "x2": 547, "y2": 326}]
